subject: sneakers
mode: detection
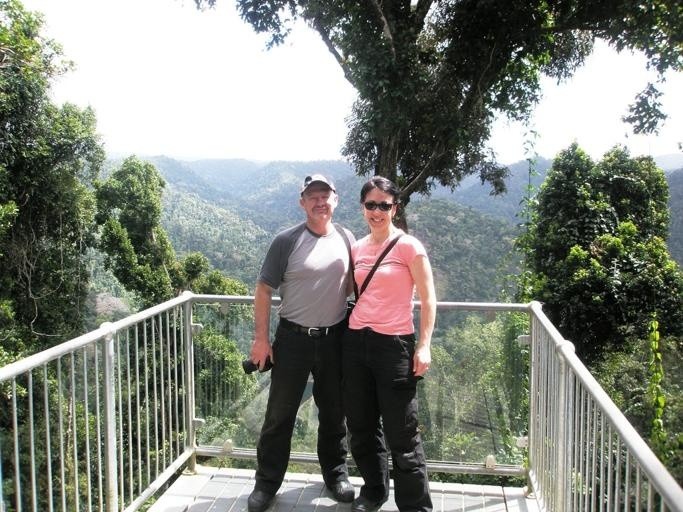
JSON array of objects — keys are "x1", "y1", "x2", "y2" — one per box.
[
  {"x1": 351, "y1": 495, "x2": 388, "y2": 512},
  {"x1": 327, "y1": 480, "x2": 355, "y2": 502},
  {"x1": 248, "y1": 490, "x2": 275, "y2": 512}
]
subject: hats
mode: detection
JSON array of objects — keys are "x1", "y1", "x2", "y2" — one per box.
[{"x1": 301, "y1": 175, "x2": 336, "y2": 196}]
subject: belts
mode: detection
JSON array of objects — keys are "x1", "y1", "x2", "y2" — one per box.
[{"x1": 280, "y1": 318, "x2": 348, "y2": 337}]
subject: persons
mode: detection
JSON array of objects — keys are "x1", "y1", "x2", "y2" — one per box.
[
  {"x1": 247, "y1": 173, "x2": 356, "y2": 511},
  {"x1": 346, "y1": 176, "x2": 438, "y2": 512}
]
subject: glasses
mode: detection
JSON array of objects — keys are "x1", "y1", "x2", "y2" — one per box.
[{"x1": 365, "y1": 200, "x2": 394, "y2": 211}]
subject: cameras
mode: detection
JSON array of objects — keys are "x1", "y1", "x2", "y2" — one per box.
[{"x1": 242, "y1": 356, "x2": 273, "y2": 374}]
